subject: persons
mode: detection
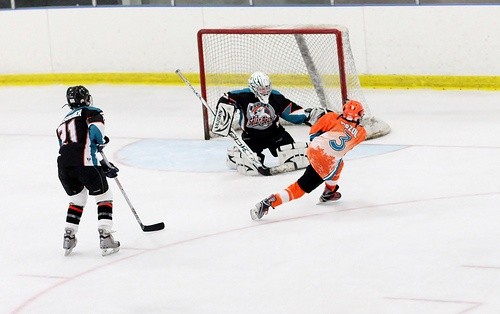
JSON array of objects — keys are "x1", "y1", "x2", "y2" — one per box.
[
  {"x1": 211, "y1": 72, "x2": 332, "y2": 174},
  {"x1": 57, "y1": 85, "x2": 120, "y2": 256},
  {"x1": 250, "y1": 99, "x2": 367, "y2": 219}
]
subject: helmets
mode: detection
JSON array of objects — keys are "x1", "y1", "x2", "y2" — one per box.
[
  {"x1": 342, "y1": 100, "x2": 364, "y2": 121},
  {"x1": 66, "y1": 85, "x2": 91, "y2": 110},
  {"x1": 248, "y1": 71, "x2": 271, "y2": 104}
]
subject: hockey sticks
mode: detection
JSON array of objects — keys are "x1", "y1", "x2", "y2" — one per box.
[
  {"x1": 176, "y1": 70, "x2": 287, "y2": 176},
  {"x1": 102, "y1": 151, "x2": 165, "y2": 232}
]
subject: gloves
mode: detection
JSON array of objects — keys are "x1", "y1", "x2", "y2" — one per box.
[{"x1": 100, "y1": 159, "x2": 119, "y2": 178}]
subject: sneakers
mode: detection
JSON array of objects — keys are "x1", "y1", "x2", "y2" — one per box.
[
  {"x1": 250, "y1": 193, "x2": 276, "y2": 221},
  {"x1": 63, "y1": 227, "x2": 78, "y2": 256},
  {"x1": 317, "y1": 184, "x2": 342, "y2": 204},
  {"x1": 98, "y1": 228, "x2": 120, "y2": 256}
]
